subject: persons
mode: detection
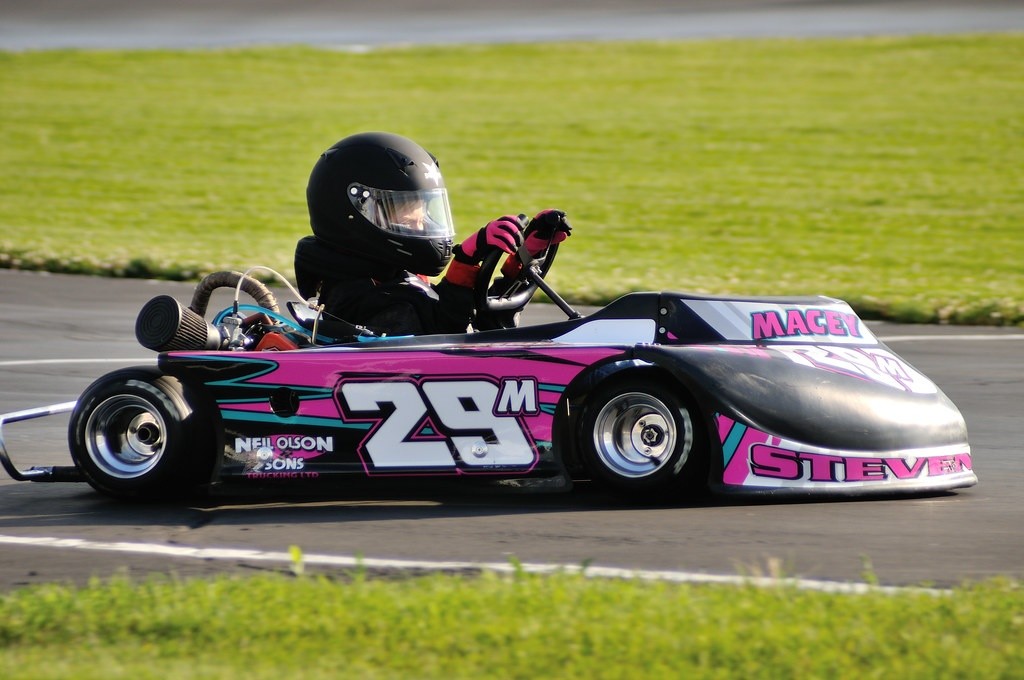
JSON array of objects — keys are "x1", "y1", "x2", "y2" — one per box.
[{"x1": 306, "y1": 130, "x2": 573, "y2": 341}]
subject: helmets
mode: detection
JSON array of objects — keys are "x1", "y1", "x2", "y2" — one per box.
[{"x1": 306, "y1": 131, "x2": 456, "y2": 277}]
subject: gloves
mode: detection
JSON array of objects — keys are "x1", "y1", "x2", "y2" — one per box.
[
  {"x1": 500, "y1": 209, "x2": 572, "y2": 281},
  {"x1": 445, "y1": 215, "x2": 525, "y2": 288}
]
